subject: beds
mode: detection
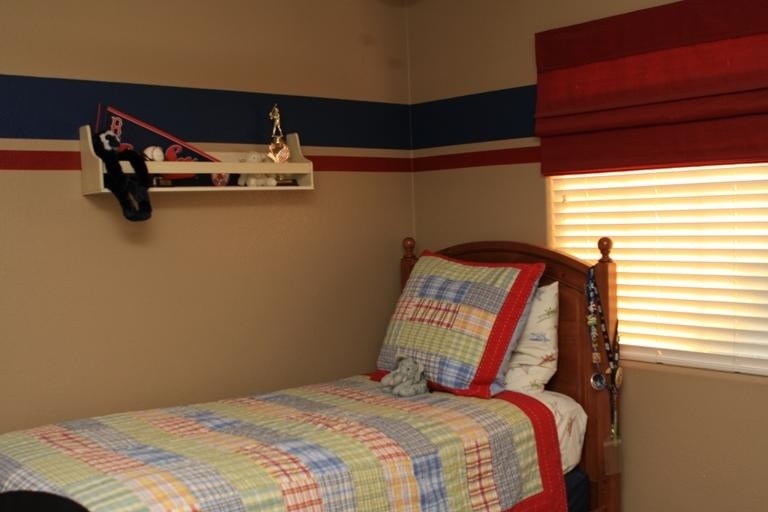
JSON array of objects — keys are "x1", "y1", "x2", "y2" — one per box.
[{"x1": 0, "y1": 236, "x2": 622, "y2": 512}]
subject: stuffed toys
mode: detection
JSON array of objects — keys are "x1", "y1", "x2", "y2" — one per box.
[
  {"x1": 378, "y1": 354, "x2": 429, "y2": 398},
  {"x1": 237, "y1": 151, "x2": 278, "y2": 187}
]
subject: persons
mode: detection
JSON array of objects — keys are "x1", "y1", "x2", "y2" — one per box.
[{"x1": 268, "y1": 103, "x2": 284, "y2": 137}]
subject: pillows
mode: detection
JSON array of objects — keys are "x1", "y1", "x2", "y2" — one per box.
[
  {"x1": 505, "y1": 281, "x2": 559, "y2": 396},
  {"x1": 370, "y1": 249, "x2": 546, "y2": 397}
]
